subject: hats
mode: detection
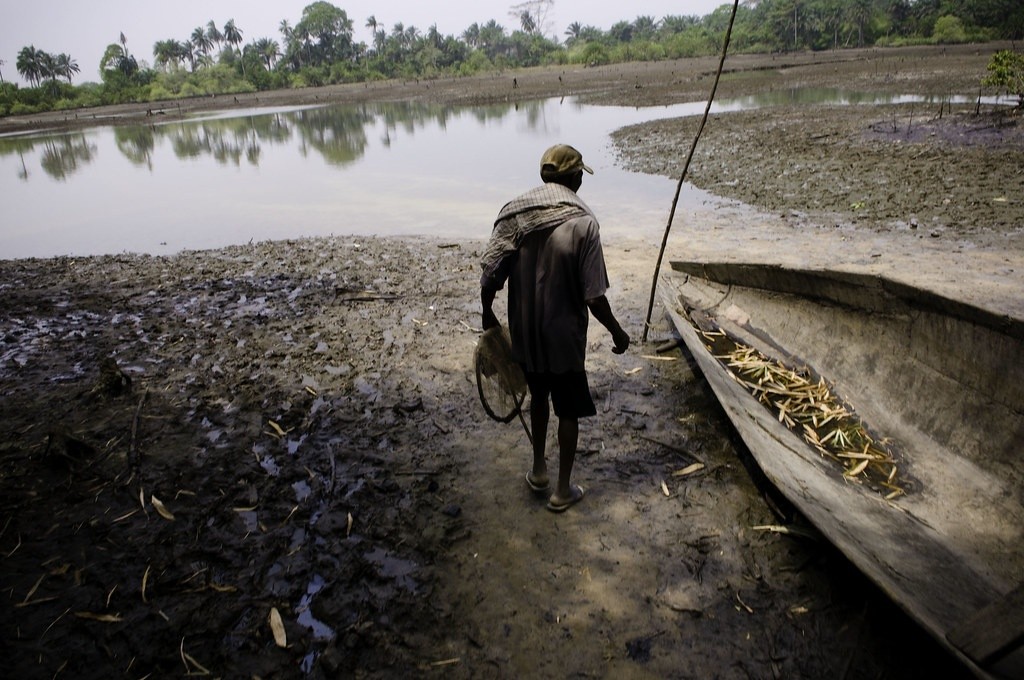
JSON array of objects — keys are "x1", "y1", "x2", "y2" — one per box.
[{"x1": 540, "y1": 144, "x2": 593, "y2": 175}]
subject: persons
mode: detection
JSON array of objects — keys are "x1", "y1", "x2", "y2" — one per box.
[{"x1": 480, "y1": 142, "x2": 630, "y2": 512}]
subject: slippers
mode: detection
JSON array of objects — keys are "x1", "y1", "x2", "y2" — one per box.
[
  {"x1": 547, "y1": 485, "x2": 585, "y2": 511},
  {"x1": 525, "y1": 470, "x2": 550, "y2": 491}
]
